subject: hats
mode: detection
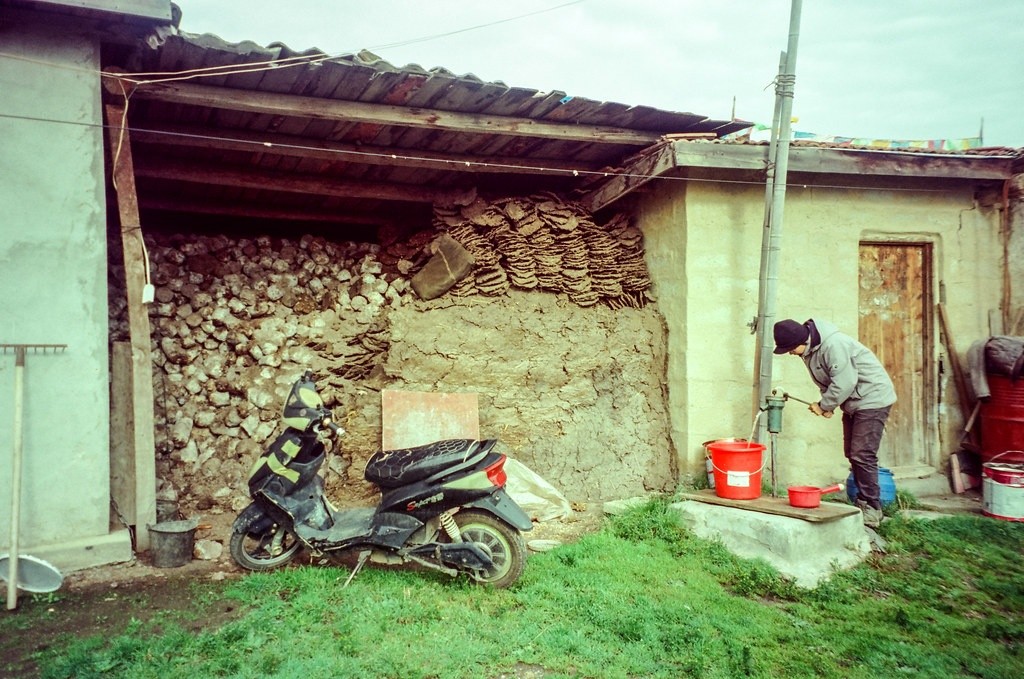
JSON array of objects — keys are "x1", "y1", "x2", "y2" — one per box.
[{"x1": 773, "y1": 318, "x2": 810, "y2": 354}]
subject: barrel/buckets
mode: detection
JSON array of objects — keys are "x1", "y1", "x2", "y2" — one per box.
[
  {"x1": 846, "y1": 463, "x2": 898, "y2": 512},
  {"x1": 708, "y1": 441, "x2": 768, "y2": 500},
  {"x1": 147, "y1": 519, "x2": 199, "y2": 570},
  {"x1": 977, "y1": 339, "x2": 1024, "y2": 478},
  {"x1": 981, "y1": 459, "x2": 1024, "y2": 522},
  {"x1": 704, "y1": 438, "x2": 747, "y2": 491}
]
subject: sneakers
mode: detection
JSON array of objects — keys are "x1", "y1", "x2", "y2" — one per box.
[{"x1": 854, "y1": 499, "x2": 883, "y2": 521}]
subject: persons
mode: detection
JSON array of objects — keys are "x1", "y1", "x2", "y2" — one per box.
[{"x1": 773, "y1": 316, "x2": 898, "y2": 522}]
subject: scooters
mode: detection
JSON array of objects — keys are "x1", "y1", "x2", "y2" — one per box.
[{"x1": 229, "y1": 370, "x2": 536, "y2": 593}]
